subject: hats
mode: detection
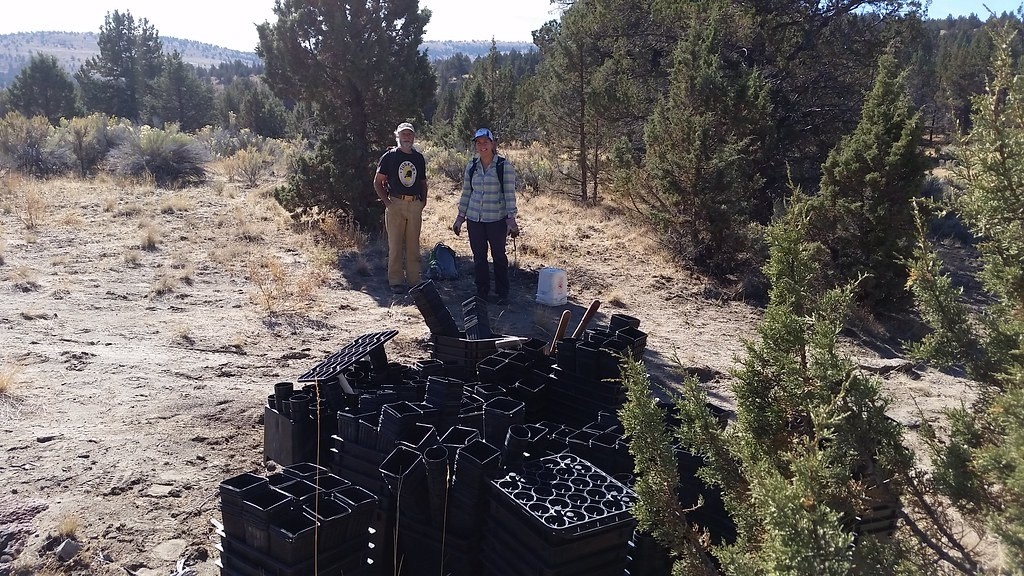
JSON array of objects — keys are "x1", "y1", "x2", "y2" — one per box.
[
  {"x1": 397, "y1": 123, "x2": 415, "y2": 133},
  {"x1": 472, "y1": 129, "x2": 494, "y2": 141}
]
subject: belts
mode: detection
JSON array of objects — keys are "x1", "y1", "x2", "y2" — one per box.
[{"x1": 390, "y1": 192, "x2": 420, "y2": 201}]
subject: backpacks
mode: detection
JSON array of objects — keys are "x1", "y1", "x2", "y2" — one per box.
[{"x1": 427, "y1": 242, "x2": 458, "y2": 279}]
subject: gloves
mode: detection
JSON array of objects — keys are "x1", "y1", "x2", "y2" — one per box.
[
  {"x1": 453, "y1": 215, "x2": 465, "y2": 235},
  {"x1": 506, "y1": 218, "x2": 520, "y2": 237}
]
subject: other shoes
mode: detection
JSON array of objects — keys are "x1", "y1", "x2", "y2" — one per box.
[
  {"x1": 474, "y1": 293, "x2": 488, "y2": 299},
  {"x1": 391, "y1": 285, "x2": 405, "y2": 293},
  {"x1": 496, "y1": 294, "x2": 510, "y2": 304}
]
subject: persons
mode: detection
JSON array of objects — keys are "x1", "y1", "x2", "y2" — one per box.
[
  {"x1": 452, "y1": 128, "x2": 520, "y2": 305},
  {"x1": 373, "y1": 123, "x2": 427, "y2": 293}
]
